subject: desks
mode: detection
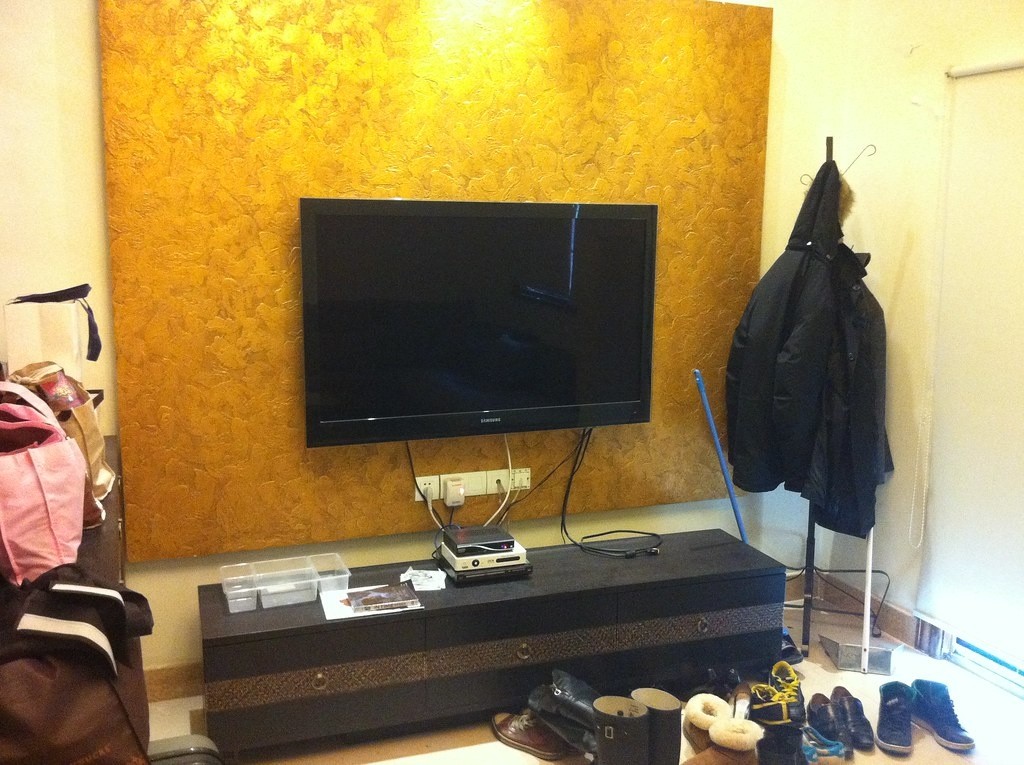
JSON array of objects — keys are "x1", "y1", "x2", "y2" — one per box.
[{"x1": 78, "y1": 435, "x2": 122, "y2": 584}]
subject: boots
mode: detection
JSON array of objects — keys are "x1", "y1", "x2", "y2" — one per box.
[
  {"x1": 592, "y1": 695, "x2": 649, "y2": 765},
  {"x1": 628, "y1": 687, "x2": 682, "y2": 765},
  {"x1": 528, "y1": 666, "x2": 608, "y2": 755},
  {"x1": 680, "y1": 718, "x2": 763, "y2": 765},
  {"x1": 682, "y1": 692, "x2": 732, "y2": 753}
]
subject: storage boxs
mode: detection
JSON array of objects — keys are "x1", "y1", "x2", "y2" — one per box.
[{"x1": 220, "y1": 553, "x2": 352, "y2": 614}]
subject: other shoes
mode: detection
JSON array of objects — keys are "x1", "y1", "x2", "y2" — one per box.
[
  {"x1": 781, "y1": 626, "x2": 804, "y2": 665},
  {"x1": 756, "y1": 725, "x2": 809, "y2": 765}
]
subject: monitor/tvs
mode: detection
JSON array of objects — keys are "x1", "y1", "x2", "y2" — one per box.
[{"x1": 300, "y1": 197, "x2": 658, "y2": 448}]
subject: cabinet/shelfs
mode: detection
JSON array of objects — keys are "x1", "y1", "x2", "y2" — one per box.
[{"x1": 198, "y1": 529, "x2": 786, "y2": 765}]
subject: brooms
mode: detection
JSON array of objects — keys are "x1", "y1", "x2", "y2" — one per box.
[{"x1": 691, "y1": 368, "x2": 800, "y2": 658}]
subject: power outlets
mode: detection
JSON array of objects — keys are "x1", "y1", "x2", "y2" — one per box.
[{"x1": 415, "y1": 475, "x2": 439, "y2": 501}]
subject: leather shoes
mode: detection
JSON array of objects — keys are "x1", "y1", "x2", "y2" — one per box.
[
  {"x1": 830, "y1": 687, "x2": 875, "y2": 750},
  {"x1": 806, "y1": 693, "x2": 854, "y2": 761}
]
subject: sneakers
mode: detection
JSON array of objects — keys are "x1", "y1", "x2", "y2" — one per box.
[
  {"x1": 492, "y1": 710, "x2": 567, "y2": 760},
  {"x1": 749, "y1": 684, "x2": 793, "y2": 726},
  {"x1": 799, "y1": 726, "x2": 845, "y2": 765},
  {"x1": 910, "y1": 680, "x2": 975, "y2": 750},
  {"x1": 769, "y1": 661, "x2": 806, "y2": 723},
  {"x1": 875, "y1": 680, "x2": 913, "y2": 753}
]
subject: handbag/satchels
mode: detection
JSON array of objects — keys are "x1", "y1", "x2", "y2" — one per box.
[
  {"x1": 1, "y1": 356, "x2": 116, "y2": 589},
  {"x1": 0, "y1": 560, "x2": 154, "y2": 765}
]
